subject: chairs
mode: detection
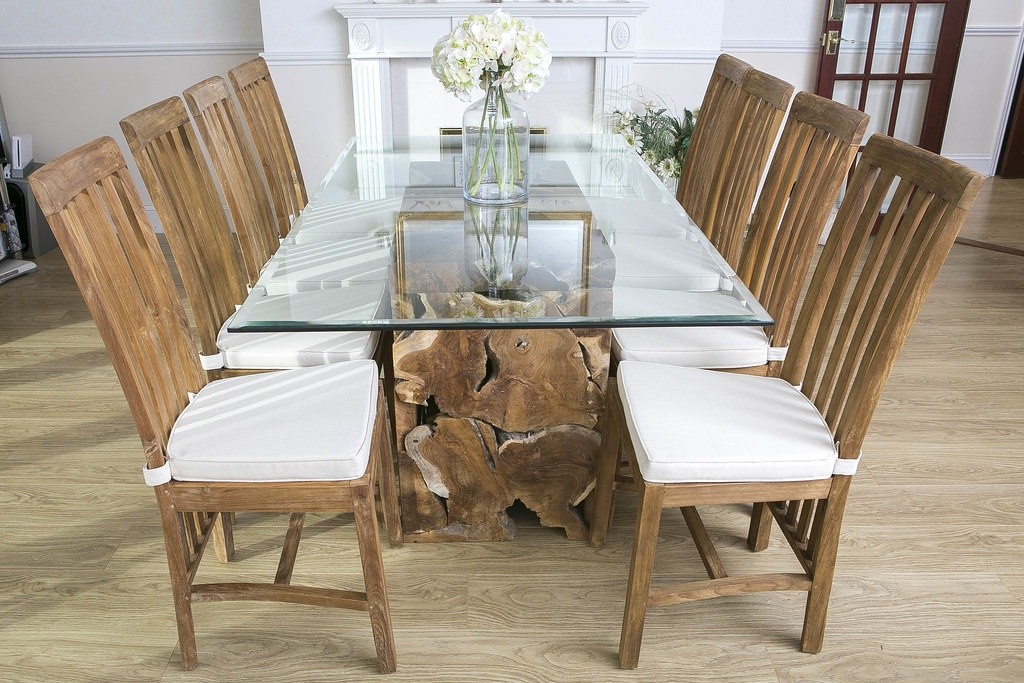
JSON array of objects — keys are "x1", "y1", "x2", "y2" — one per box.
[
  {"x1": 538, "y1": 51, "x2": 986, "y2": 671},
  {"x1": 27, "y1": 55, "x2": 403, "y2": 675}
]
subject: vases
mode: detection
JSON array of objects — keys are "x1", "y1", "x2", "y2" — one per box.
[
  {"x1": 463, "y1": 197, "x2": 534, "y2": 303},
  {"x1": 461, "y1": 66, "x2": 531, "y2": 202}
]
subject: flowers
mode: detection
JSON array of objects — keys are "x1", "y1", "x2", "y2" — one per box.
[
  {"x1": 428, "y1": 6, "x2": 552, "y2": 102},
  {"x1": 586, "y1": 79, "x2": 701, "y2": 189}
]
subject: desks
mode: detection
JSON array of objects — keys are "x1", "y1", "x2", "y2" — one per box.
[{"x1": 226, "y1": 136, "x2": 778, "y2": 545}]
